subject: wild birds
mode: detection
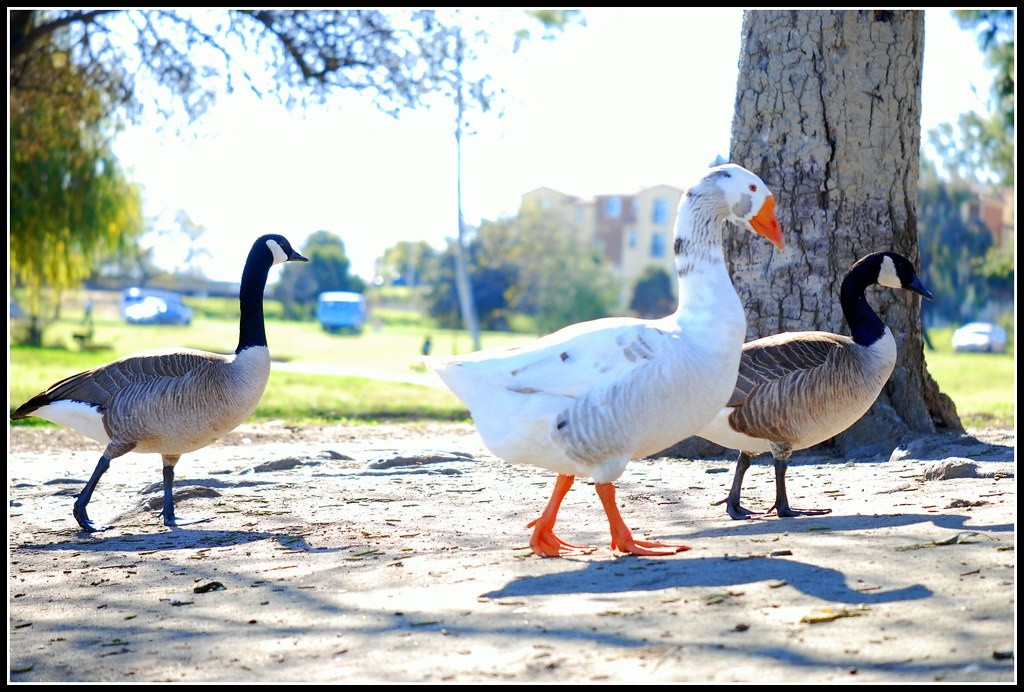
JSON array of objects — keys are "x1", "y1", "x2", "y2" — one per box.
[
  {"x1": 694, "y1": 249, "x2": 937, "y2": 520},
  {"x1": 432, "y1": 164, "x2": 786, "y2": 561},
  {"x1": 9, "y1": 234, "x2": 313, "y2": 535}
]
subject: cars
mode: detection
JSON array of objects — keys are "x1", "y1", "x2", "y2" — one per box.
[
  {"x1": 123, "y1": 297, "x2": 190, "y2": 325},
  {"x1": 952, "y1": 321, "x2": 1006, "y2": 355}
]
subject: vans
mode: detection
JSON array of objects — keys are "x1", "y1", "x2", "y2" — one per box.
[{"x1": 317, "y1": 287, "x2": 364, "y2": 334}]
